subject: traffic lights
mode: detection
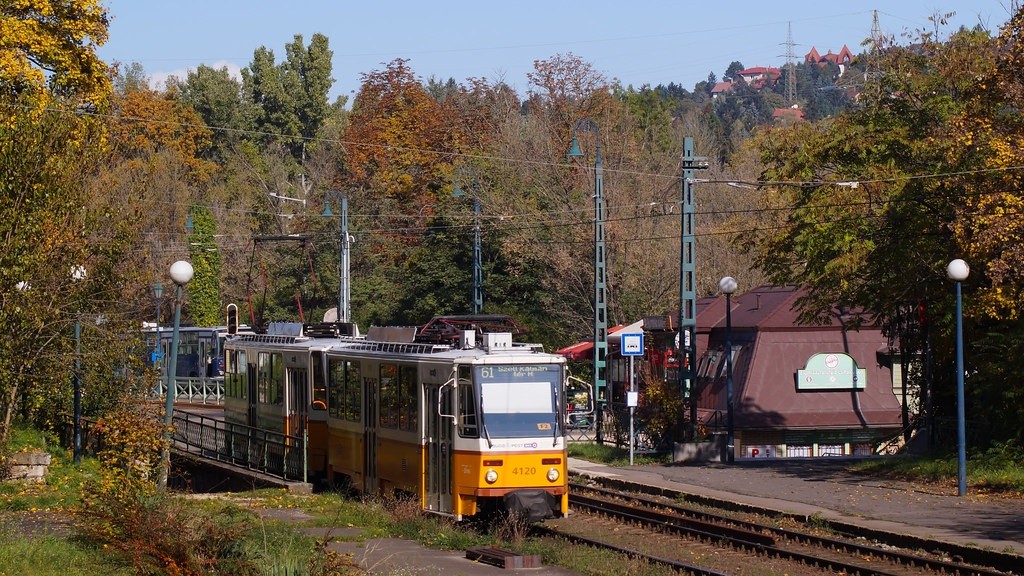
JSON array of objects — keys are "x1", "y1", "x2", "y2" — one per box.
[{"x1": 226, "y1": 304, "x2": 238, "y2": 336}]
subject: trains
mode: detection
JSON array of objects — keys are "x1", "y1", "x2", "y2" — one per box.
[
  {"x1": 221, "y1": 330, "x2": 594, "y2": 528},
  {"x1": 118, "y1": 326, "x2": 256, "y2": 395}
]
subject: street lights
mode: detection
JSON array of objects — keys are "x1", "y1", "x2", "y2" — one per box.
[
  {"x1": 15, "y1": 281, "x2": 33, "y2": 419},
  {"x1": 718, "y1": 277, "x2": 738, "y2": 468},
  {"x1": 69, "y1": 265, "x2": 89, "y2": 464},
  {"x1": 157, "y1": 260, "x2": 193, "y2": 486},
  {"x1": 949, "y1": 259, "x2": 970, "y2": 500}
]
